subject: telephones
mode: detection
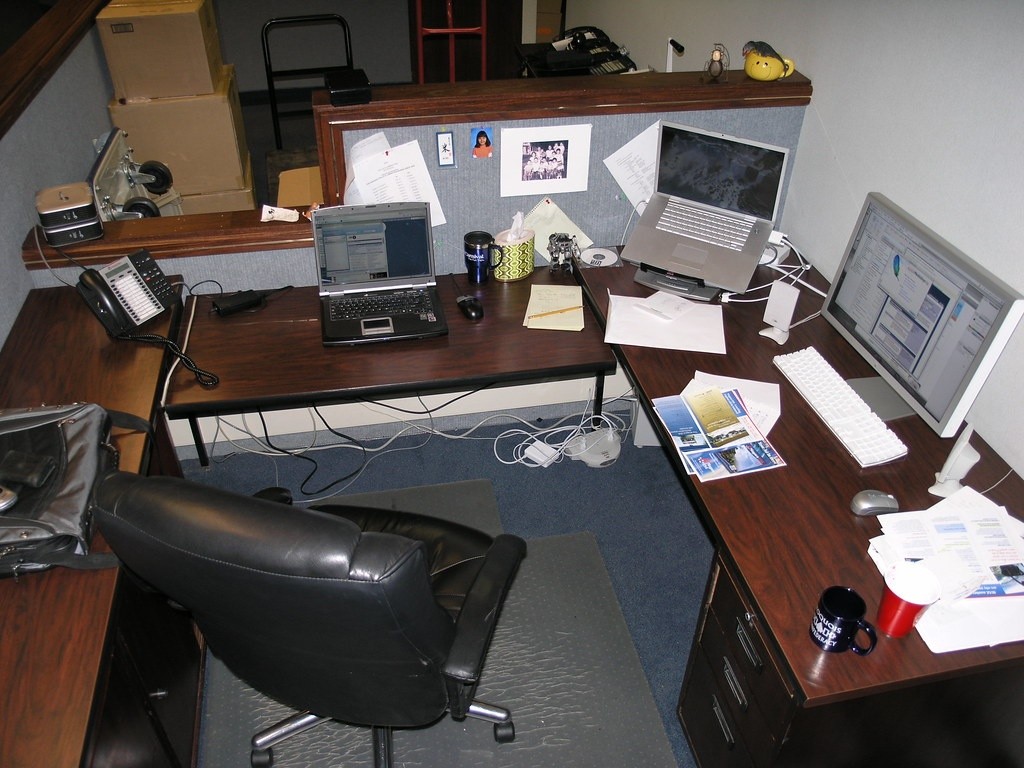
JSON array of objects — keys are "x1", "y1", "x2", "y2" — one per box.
[
  {"x1": 75, "y1": 252, "x2": 180, "y2": 341},
  {"x1": 555, "y1": 25, "x2": 610, "y2": 51}
]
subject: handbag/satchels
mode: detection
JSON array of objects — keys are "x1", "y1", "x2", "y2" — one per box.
[{"x1": 0, "y1": 403, "x2": 120, "y2": 577}]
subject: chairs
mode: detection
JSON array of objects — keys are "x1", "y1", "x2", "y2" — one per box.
[
  {"x1": 83, "y1": 471, "x2": 526, "y2": 768},
  {"x1": 261, "y1": 13, "x2": 355, "y2": 149}
]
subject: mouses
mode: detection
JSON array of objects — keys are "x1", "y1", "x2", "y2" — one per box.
[
  {"x1": 849, "y1": 490, "x2": 899, "y2": 516},
  {"x1": 456, "y1": 295, "x2": 483, "y2": 321}
]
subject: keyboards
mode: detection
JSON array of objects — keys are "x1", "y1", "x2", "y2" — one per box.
[{"x1": 774, "y1": 346, "x2": 908, "y2": 468}]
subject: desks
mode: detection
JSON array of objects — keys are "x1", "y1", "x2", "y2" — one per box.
[
  {"x1": 1, "y1": 238, "x2": 1024, "y2": 768},
  {"x1": 517, "y1": 43, "x2": 592, "y2": 78}
]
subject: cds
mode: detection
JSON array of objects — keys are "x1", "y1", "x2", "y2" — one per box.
[{"x1": 581, "y1": 248, "x2": 618, "y2": 266}]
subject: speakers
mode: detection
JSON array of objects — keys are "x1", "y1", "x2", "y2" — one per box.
[
  {"x1": 758, "y1": 280, "x2": 800, "y2": 345},
  {"x1": 928, "y1": 424, "x2": 981, "y2": 497}
]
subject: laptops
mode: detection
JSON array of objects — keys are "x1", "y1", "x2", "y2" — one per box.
[
  {"x1": 311, "y1": 201, "x2": 449, "y2": 348},
  {"x1": 620, "y1": 119, "x2": 789, "y2": 295}
]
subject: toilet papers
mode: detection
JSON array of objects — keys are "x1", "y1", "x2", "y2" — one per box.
[{"x1": 491, "y1": 211, "x2": 537, "y2": 282}]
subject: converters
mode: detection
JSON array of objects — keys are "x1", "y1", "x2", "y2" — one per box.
[{"x1": 213, "y1": 288, "x2": 262, "y2": 318}]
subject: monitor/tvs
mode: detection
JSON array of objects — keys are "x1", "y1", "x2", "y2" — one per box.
[{"x1": 820, "y1": 191, "x2": 1024, "y2": 438}]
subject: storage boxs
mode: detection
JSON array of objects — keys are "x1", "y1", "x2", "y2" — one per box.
[
  {"x1": 536, "y1": 1, "x2": 563, "y2": 43},
  {"x1": 105, "y1": 63, "x2": 247, "y2": 196},
  {"x1": 175, "y1": 151, "x2": 257, "y2": 214},
  {"x1": 97, "y1": 0, "x2": 223, "y2": 99}
]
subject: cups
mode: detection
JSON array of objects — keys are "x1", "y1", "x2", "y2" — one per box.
[
  {"x1": 876, "y1": 563, "x2": 941, "y2": 639},
  {"x1": 809, "y1": 585, "x2": 878, "y2": 657},
  {"x1": 464, "y1": 231, "x2": 503, "y2": 286},
  {"x1": 493, "y1": 228, "x2": 536, "y2": 283},
  {"x1": 744, "y1": 50, "x2": 795, "y2": 82}
]
